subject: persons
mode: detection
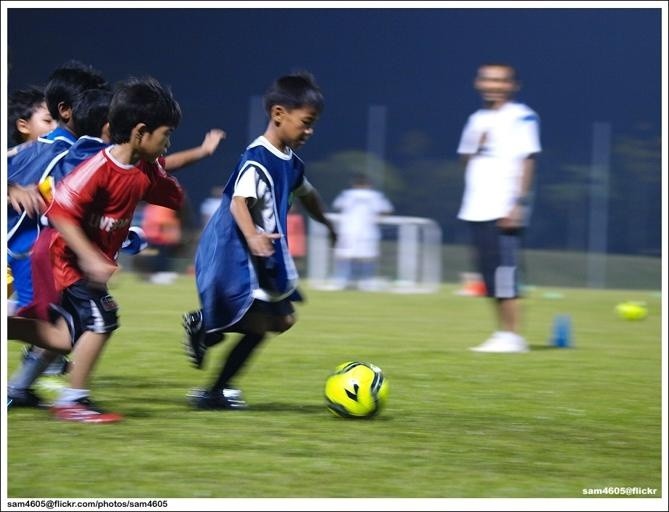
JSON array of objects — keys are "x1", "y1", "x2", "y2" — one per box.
[
  {"x1": 7, "y1": 75, "x2": 195, "y2": 424},
  {"x1": 181, "y1": 70, "x2": 344, "y2": 412},
  {"x1": 7, "y1": 82, "x2": 59, "y2": 219},
  {"x1": 450, "y1": 61, "x2": 544, "y2": 355},
  {"x1": 6, "y1": 63, "x2": 113, "y2": 375},
  {"x1": 7, "y1": 85, "x2": 229, "y2": 412},
  {"x1": 139, "y1": 199, "x2": 185, "y2": 282},
  {"x1": 199, "y1": 182, "x2": 229, "y2": 230},
  {"x1": 329, "y1": 174, "x2": 395, "y2": 292}
]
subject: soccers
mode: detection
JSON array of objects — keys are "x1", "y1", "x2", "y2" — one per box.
[
  {"x1": 616, "y1": 295, "x2": 646, "y2": 326},
  {"x1": 323, "y1": 360, "x2": 389, "y2": 418}
]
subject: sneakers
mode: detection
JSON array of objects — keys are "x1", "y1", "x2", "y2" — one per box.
[
  {"x1": 8, "y1": 345, "x2": 127, "y2": 424},
  {"x1": 187, "y1": 385, "x2": 246, "y2": 411},
  {"x1": 470, "y1": 330, "x2": 531, "y2": 354},
  {"x1": 181, "y1": 308, "x2": 224, "y2": 370}
]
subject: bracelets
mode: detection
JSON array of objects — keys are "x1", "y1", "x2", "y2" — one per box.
[{"x1": 516, "y1": 196, "x2": 530, "y2": 207}]
